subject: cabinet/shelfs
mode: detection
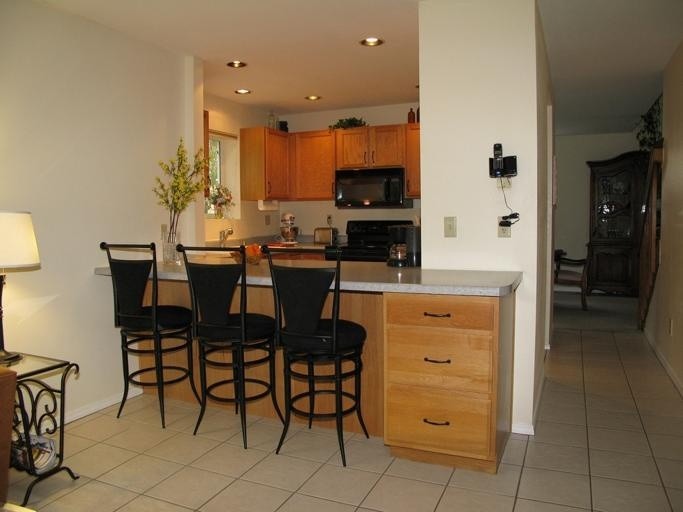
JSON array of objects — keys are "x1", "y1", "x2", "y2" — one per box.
[
  {"x1": 240, "y1": 128, "x2": 290, "y2": 201},
  {"x1": 294, "y1": 132, "x2": 336, "y2": 201},
  {"x1": 585, "y1": 149, "x2": 655, "y2": 296},
  {"x1": 337, "y1": 123, "x2": 408, "y2": 170},
  {"x1": 403, "y1": 125, "x2": 420, "y2": 198},
  {"x1": 264, "y1": 251, "x2": 326, "y2": 261},
  {"x1": 382, "y1": 293, "x2": 512, "y2": 475}
]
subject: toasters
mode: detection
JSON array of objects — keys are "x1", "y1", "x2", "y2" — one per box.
[{"x1": 313, "y1": 227, "x2": 336, "y2": 244}]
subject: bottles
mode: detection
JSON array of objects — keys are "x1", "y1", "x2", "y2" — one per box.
[{"x1": 407, "y1": 107, "x2": 414, "y2": 122}]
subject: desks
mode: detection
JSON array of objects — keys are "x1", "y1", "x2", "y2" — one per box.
[{"x1": 0, "y1": 346, "x2": 79, "y2": 510}]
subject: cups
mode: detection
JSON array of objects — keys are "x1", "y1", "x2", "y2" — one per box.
[{"x1": 405, "y1": 224, "x2": 420, "y2": 265}]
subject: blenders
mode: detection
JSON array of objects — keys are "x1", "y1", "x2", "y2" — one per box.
[{"x1": 279, "y1": 213, "x2": 298, "y2": 245}]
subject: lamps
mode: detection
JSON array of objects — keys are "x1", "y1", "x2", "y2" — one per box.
[{"x1": 0, "y1": 209, "x2": 41, "y2": 367}]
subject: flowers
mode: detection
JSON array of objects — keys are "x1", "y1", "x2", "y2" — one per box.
[
  {"x1": 205, "y1": 179, "x2": 235, "y2": 213},
  {"x1": 152, "y1": 132, "x2": 215, "y2": 241}
]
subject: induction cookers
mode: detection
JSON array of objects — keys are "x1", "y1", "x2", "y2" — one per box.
[{"x1": 326, "y1": 240, "x2": 406, "y2": 261}]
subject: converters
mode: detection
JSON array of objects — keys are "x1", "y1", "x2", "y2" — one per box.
[{"x1": 500, "y1": 221, "x2": 511, "y2": 227}]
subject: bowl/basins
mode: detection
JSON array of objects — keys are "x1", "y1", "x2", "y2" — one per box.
[{"x1": 231, "y1": 252, "x2": 262, "y2": 266}]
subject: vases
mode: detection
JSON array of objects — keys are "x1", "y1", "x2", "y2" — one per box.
[
  {"x1": 214, "y1": 207, "x2": 224, "y2": 220},
  {"x1": 161, "y1": 239, "x2": 178, "y2": 263}
]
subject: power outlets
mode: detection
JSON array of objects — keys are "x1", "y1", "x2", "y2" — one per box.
[{"x1": 498, "y1": 225, "x2": 511, "y2": 238}]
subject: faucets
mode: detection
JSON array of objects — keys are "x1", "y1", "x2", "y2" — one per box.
[{"x1": 220, "y1": 228, "x2": 233, "y2": 247}]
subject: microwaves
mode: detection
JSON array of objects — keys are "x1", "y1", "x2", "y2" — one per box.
[{"x1": 333, "y1": 166, "x2": 414, "y2": 209}]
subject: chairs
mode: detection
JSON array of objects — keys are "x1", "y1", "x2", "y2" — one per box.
[
  {"x1": 552, "y1": 256, "x2": 590, "y2": 309},
  {"x1": 251, "y1": 243, "x2": 370, "y2": 468},
  {"x1": 96, "y1": 239, "x2": 204, "y2": 426},
  {"x1": 166, "y1": 241, "x2": 289, "y2": 452}
]
